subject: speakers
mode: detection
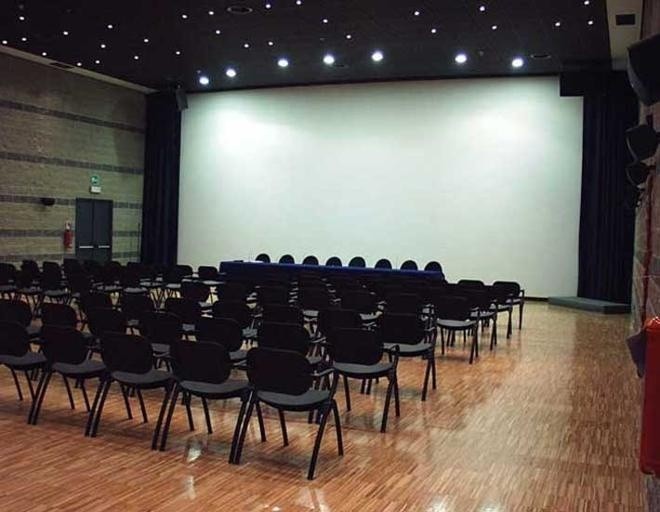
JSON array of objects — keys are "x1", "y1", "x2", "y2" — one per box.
[
  {"x1": 626, "y1": 34, "x2": 660, "y2": 105},
  {"x1": 624, "y1": 162, "x2": 650, "y2": 184},
  {"x1": 146, "y1": 86, "x2": 188, "y2": 117},
  {"x1": 625, "y1": 126, "x2": 660, "y2": 160}
]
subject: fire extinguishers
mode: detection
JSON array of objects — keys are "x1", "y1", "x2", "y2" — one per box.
[{"x1": 64, "y1": 220, "x2": 73, "y2": 249}]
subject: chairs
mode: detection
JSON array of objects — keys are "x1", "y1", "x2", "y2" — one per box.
[
  {"x1": 374, "y1": 251, "x2": 527, "y2": 402},
  {"x1": 2, "y1": 251, "x2": 400, "y2": 477}
]
